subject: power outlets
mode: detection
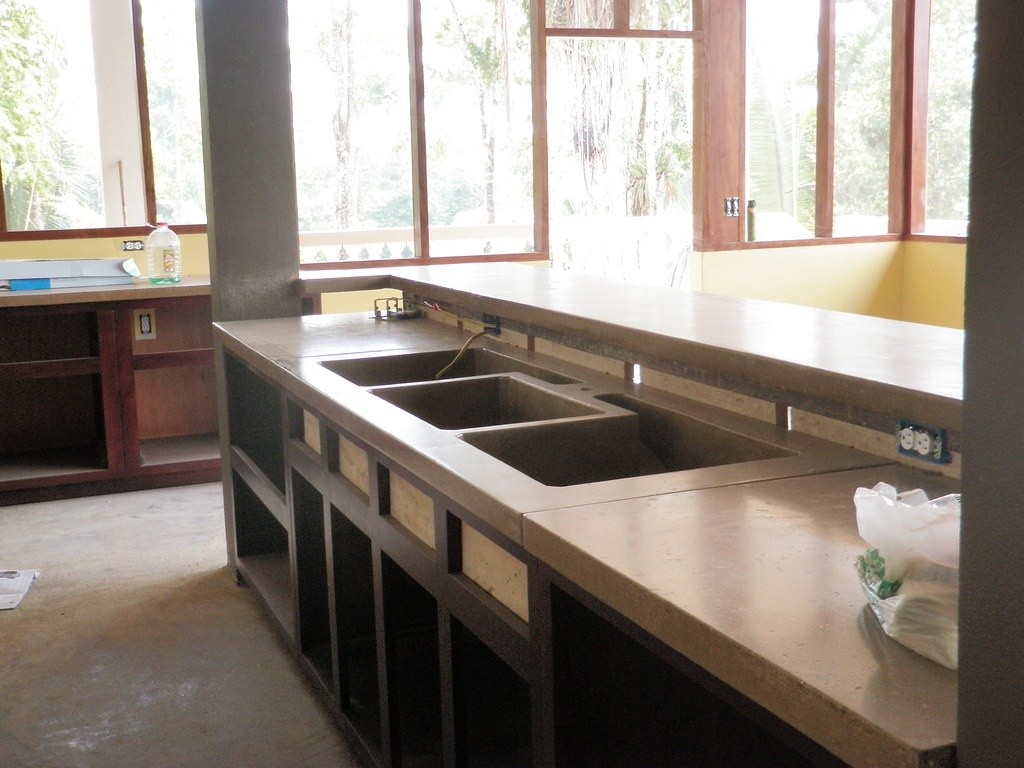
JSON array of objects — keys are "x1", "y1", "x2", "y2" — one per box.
[
  {"x1": 481, "y1": 313, "x2": 501, "y2": 335},
  {"x1": 122, "y1": 240, "x2": 145, "y2": 251},
  {"x1": 895, "y1": 417, "x2": 954, "y2": 464},
  {"x1": 134, "y1": 308, "x2": 157, "y2": 341}
]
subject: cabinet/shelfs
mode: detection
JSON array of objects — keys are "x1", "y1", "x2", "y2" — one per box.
[
  {"x1": 0, "y1": 292, "x2": 321, "y2": 508},
  {"x1": 210, "y1": 344, "x2": 852, "y2": 768}
]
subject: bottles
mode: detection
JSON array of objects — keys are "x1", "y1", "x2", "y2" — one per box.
[{"x1": 146, "y1": 222, "x2": 186, "y2": 286}]
